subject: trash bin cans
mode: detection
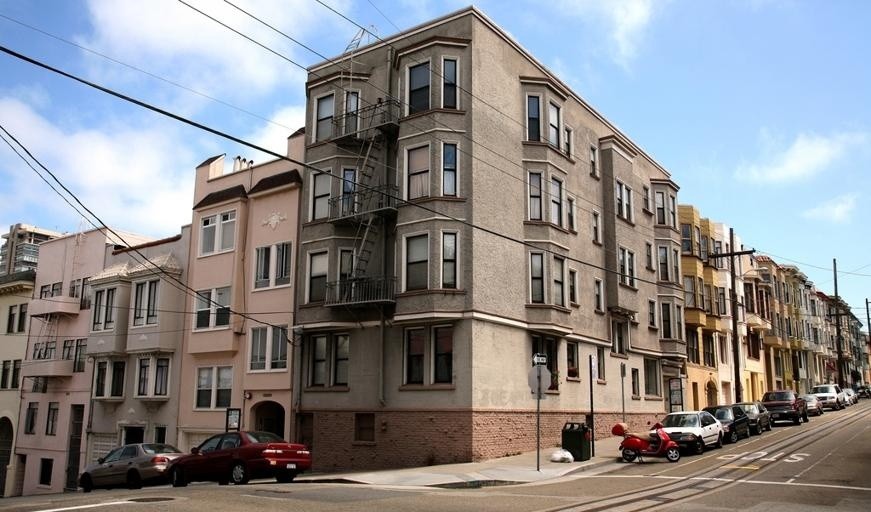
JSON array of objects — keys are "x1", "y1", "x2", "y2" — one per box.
[{"x1": 562, "y1": 422, "x2": 592, "y2": 461}]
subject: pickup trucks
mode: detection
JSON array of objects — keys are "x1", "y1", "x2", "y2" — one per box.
[{"x1": 760, "y1": 389, "x2": 809, "y2": 425}]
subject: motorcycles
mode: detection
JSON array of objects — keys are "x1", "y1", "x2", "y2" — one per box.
[{"x1": 611, "y1": 412, "x2": 681, "y2": 466}]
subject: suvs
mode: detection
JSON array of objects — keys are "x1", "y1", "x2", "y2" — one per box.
[{"x1": 810, "y1": 384, "x2": 846, "y2": 411}]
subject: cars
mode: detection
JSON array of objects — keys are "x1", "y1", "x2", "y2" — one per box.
[
  {"x1": 797, "y1": 393, "x2": 824, "y2": 417},
  {"x1": 76, "y1": 443, "x2": 190, "y2": 494},
  {"x1": 164, "y1": 430, "x2": 312, "y2": 488},
  {"x1": 647, "y1": 401, "x2": 771, "y2": 456}
]
